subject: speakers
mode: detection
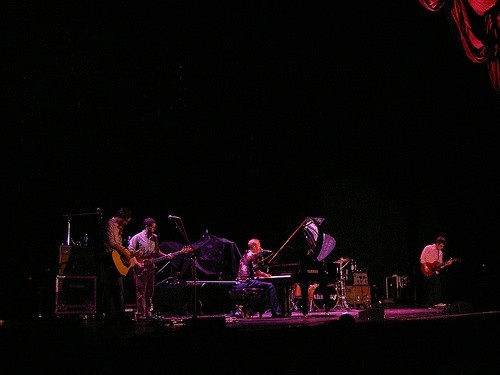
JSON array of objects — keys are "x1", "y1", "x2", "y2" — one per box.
[
  {"x1": 344, "y1": 272, "x2": 371, "y2": 305},
  {"x1": 58, "y1": 245, "x2": 95, "y2": 276}
]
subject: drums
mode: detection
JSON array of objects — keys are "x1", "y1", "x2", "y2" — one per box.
[{"x1": 308, "y1": 283, "x2": 340, "y2": 311}]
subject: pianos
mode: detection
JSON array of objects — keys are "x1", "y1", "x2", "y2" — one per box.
[{"x1": 256, "y1": 262, "x2": 300, "y2": 316}]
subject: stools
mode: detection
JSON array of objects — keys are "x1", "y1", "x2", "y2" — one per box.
[{"x1": 230, "y1": 287, "x2": 263, "y2": 318}]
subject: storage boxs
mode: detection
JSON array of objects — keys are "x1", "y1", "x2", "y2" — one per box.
[{"x1": 54, "y1": 274, "x2": 97, "y2": 315}]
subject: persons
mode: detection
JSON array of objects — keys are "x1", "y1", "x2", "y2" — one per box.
[
  {"x1": 98, "y1": 208, "x2": 130, "y2": 313},
  {"x1": 127, "y1": 217, "x2": 174, "y2": 316},
  {"x1": 238, "y1": 236, "x2": 285, "y2": 317},
  {"x1": 303, "y1": 213, "x2": 331, "y2": 313},
  {"x1": 418, "y1": 234, "x2": 452, "y2": 308}
]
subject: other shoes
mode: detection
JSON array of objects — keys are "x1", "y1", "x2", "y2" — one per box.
[
  {"x1": 271, "y1": 313, "x2": 283, "y2": 318},
  {"x1": 137, "y1": 311, "x2": 153, "y2": 320}
]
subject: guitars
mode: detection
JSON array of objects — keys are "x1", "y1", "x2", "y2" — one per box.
[
  {"x1": 420, "y1": 257, "x2": 459, "y2": 277},
  {"x1": 112, "y1": 247, "x2": 141, "y2": 276},
  {"x1": 134, "y1": 245, "x2": 193, "y2": 279}
]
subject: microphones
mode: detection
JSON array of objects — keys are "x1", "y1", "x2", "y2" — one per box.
[
  {"x1": 168, "y1": 215, "x2": 180, "y2": 220},
  {"x1": 96, "y1": 208, "x2": 103, "y2": 216}
]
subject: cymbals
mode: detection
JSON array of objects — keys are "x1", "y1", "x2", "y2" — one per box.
[{"x1": 333, "y1": 258, "x2": 350, "y2": 263}]
